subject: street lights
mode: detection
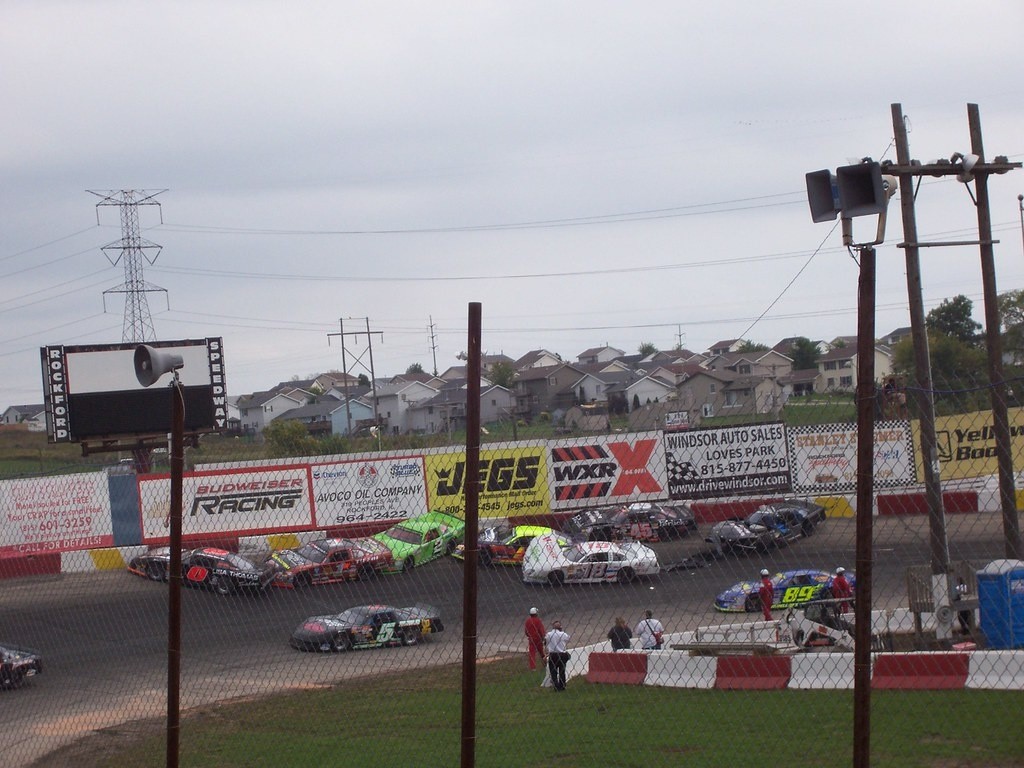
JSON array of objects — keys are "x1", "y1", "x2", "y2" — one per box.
[{"x1": 804, "y1": 159, "x2": 898, "y2": 768}]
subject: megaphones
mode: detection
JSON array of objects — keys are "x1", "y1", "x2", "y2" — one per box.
[
  {"x1": 836, "y1": 162, "x2": 897, "y2": 220},
  {"x1": 134, "y1": 344, "x2": 184, "y2": 387},
  {"x1": 806, "y1": 169, "x2": 841, "y2": 223}
]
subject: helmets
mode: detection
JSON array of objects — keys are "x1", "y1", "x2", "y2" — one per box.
[
  {"x1": 760, "y1": 569, "x2": 769, "y2": 576},
  {"x1": 836, "y1": 567, "x2": 845, "y2": 574},
  {"x1": 529, "y1": 607, "x2": 538, "y2": 616}
]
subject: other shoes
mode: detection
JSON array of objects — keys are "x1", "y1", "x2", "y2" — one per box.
[
  {"x1": 531, "y1": 668, "x2": 536, "y2": 672},
  {"x1": 554, "y1": 684, "x2": 566, "y2": 691}
]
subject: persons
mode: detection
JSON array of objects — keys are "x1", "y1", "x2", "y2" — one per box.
[
  {"x1": 834, "y1": 567, "x2": 853, "y2": 614},
  {"x1": 524, "y1": 607, "x2": 547, "y2": 670},
  {"x1": 635, "y1": 609, "x2": 664, "y2": 649},
  {"x1": 758, "y1": 568, "x2": 774, "y2": 621},
  {"x1": 544, "y1": 621, "x2": 570, "y2": 692},
  {"x1": 956, "y1": 576, "x2": 974, "y2": 634},
  {"x1": 606, "y1": 617, "x2": 632, "y2": 651}
]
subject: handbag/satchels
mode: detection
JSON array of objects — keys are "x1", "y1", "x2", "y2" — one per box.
[{"x1": 655, "y1": 632, "x2": 664, "y2": 644}]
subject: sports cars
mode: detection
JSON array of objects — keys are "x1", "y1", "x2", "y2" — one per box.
[
  {"x1": 450, "y1": 523, "x2": 572, "y2": 567},
  {"x1": 0, "y1": 643, "x2": 43, "y2": 690},
  {"x1": 521, "y1": 529, "x2": 660, "y2": 586},
  {"x1": 704, "y1": 498, "x2": 826, "y2": 557},
  {"x1": 127, "y1": 543, "x2": 280, "y2": 596},
  {"x1": 264, "y1": 536, "x2": 396, "y2": 591},
  {"x1": 562, "y1": 501, "x2": 699, "y2": 544},
  {"x1": 372, "y1": 508, "x2": 466, "y2": 577},
  {"x1": 288, "y1": 599, "x2": 445, "y2": 653},
  {"x1": 712, "y1": 570, "x2": 857, "y2": 612}
]
